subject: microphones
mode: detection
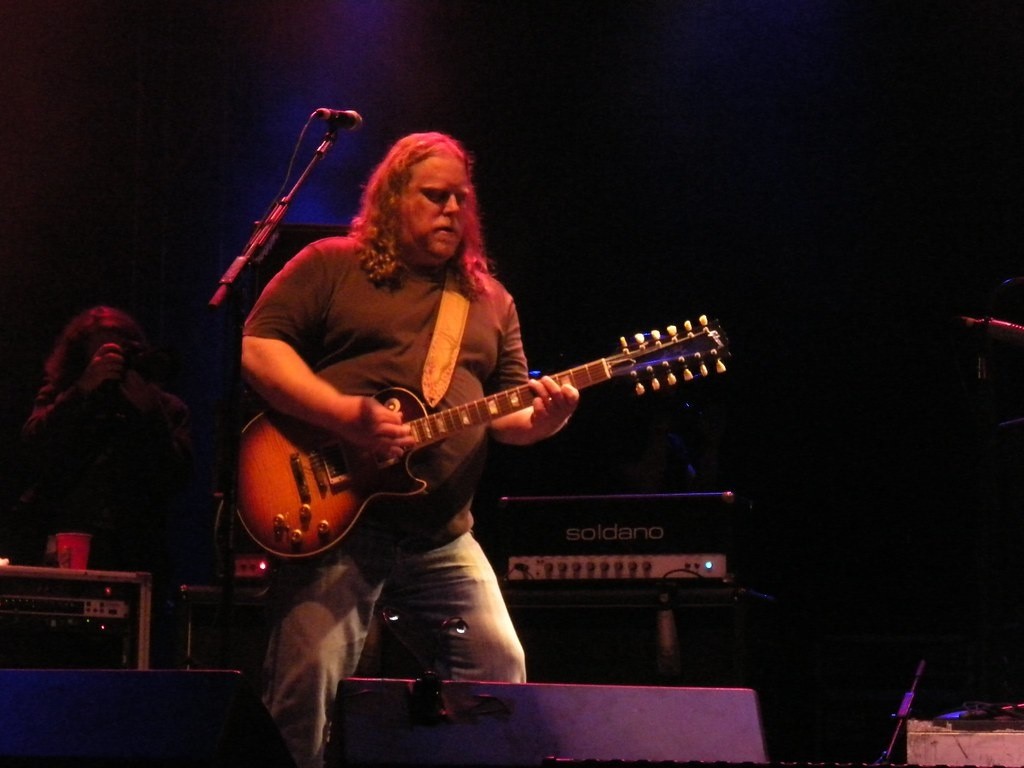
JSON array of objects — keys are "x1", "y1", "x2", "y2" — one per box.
[
  {"x1": 961, "y1": 315, "x2": 1024, "y2": 346},
  {"x1": 316, "y1": 108, "x2": 363, "y2": 131},
  {"x1": 659, "y1": 585, "x2": 681, "y2": 679}
]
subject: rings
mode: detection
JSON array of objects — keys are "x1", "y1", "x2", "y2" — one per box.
[{"x1": 545, "y1": 397, "x2": 555, "y2": 409}]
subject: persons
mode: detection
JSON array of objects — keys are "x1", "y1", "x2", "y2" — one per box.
[
  {"x1": 241, "y1": 133, "x2": 581, "y2": 768},
  {"x1": 19, "y1": 305, "x2": 199, "y2": 669}
]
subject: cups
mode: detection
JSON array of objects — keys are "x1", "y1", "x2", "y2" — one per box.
[{"x1": 56, "y1": 533, "x2": 91, "y2": 569}]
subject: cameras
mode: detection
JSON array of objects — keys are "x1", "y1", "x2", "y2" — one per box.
[{"x1": 114, "y1": 341, "x2": 149, "y2": 379}]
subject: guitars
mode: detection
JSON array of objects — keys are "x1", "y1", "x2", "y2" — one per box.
[{"x1": 232, "y1": 313, "x2": 731, "y2": 559}]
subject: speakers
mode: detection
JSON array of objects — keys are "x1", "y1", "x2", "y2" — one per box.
[
  {"x1": 985, "y1": 279, "x2": 1024, "y2": 705},
  {"x1": 0, "y1": 668, "x2": 301, "y2": 768},
  {"x1": 304, "y1": 676, "x2": 769, "y2": 768}
]
